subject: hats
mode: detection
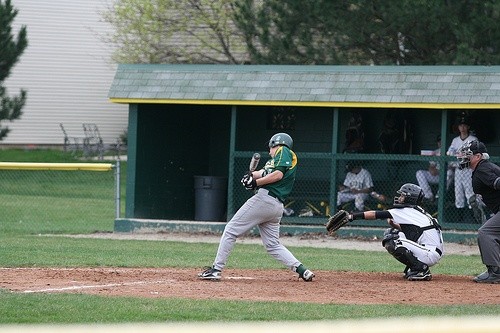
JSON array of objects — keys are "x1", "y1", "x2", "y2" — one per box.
[
  {"x1": 344, "y1": 160, "x2": 359, "y2": 173},
  {"x1": 462, "y1": 140, "x2": 489, "y2": 155},
  {"x1": 456, "y1": 111, "x2": 469, "y2": 123}
]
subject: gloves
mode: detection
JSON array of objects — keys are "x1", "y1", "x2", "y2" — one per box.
[
  {"x1": 245, "y1": 179, "x2": 258, "y2": 191},
  {"x1": 240, "y1": 171, "x2": 253, "y2": 186}
]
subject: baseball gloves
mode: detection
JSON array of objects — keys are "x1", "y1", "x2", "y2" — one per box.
[{"x1": 325, "y1": 209, "x2": 348, "y2": 232}]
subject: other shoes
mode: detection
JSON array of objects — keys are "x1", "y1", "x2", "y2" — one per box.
[{"x1": 298, "y1": 209, "x2": 313, "y2": 217}]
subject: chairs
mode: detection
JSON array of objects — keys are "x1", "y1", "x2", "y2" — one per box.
[{"x1": 60, "y1": 121, "x2": 122, "y2": 161}]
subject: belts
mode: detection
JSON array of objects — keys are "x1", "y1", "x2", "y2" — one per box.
[
  {"x1": 256, "y1": 187, "x2": 284, "y2": 203},
  {"x1": 419, "y1": 244, "x2": 442, "y2": 256}
]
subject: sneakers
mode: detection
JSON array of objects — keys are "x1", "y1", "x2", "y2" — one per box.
[
  {"x1": 298, "y1": 268, "x2": 315, "y2": 282},
  {"x1": 473, "y1": 267, "x2": 500, "y2": 284},
  {"x1": 408, "y1": 269, "x2": 432, "y2": 280},
  {"x1": 198, "y1": 266, "x2": 222, "y2": 280}
]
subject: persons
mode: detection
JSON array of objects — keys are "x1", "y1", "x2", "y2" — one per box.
[
  {"x1": 337, "y1": 160, "x2": 374, "y2": 212},
  {"x1": 198, "y1": 133, "x2": 315, "y2": 282},
  {"x1": 456, "y1": 139, "x2": 500, "y2": 284},
  {"x1": 326, "y1": 184, "x2": 444, "y2": 280},
  {"x1": 417, "y1": 115, "x2": 482, "y2": 223}
]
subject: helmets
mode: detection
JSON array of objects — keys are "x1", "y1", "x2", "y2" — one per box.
[
  {"x1": 269, "y1": 133, "x2": 293, "y2": 149},
  {"x1": 397, "y1": 183, "x2": 423, "y2": 205}
]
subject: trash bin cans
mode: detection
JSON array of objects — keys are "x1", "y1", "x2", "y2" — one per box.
[{"x1": 194, "y1": 177, "x2": 227, "y2": 220}]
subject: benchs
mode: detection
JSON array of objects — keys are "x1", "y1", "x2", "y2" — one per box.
[{"x1": 285, "y1": 192, "x2": 490, "y2": 222}]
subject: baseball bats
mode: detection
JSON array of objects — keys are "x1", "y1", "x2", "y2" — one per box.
[{"x1": 246, "y1": 153, "x2": 261, "y2": 184}]
